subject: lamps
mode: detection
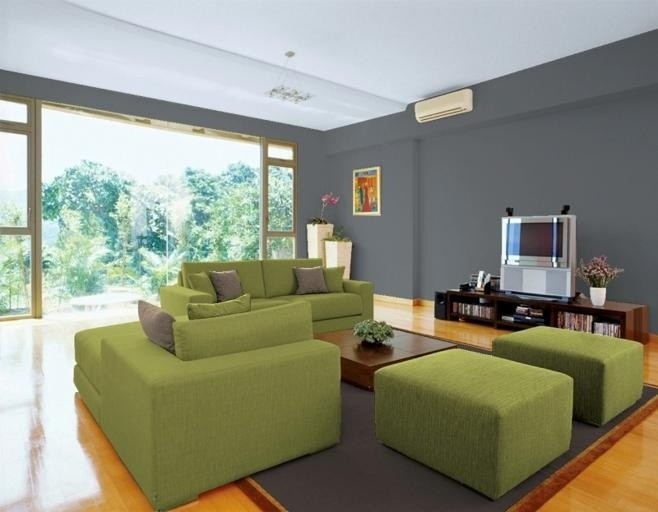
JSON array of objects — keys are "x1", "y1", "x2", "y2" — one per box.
[{"x1": 268, "y1": 51, "x2": 314, "y2": 106}]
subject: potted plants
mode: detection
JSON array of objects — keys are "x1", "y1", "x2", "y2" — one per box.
[
  {"x1": 351, "y1": 319, "x2": 394, "y2": 349},
  {"x1": 323, "y1": 226, "x2": 353, "y2": 280}
]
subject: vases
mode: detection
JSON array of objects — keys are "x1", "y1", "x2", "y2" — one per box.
[{"x1": 307, "y1": 223, "x2": 333, "y2": 258}]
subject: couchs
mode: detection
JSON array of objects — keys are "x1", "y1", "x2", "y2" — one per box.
[
  {"x1": 74, "y1": 301, "x2": 342, "y2": 512},
  {"x1": 159, "y1": 258, "x2": 374, "y2": 330}
]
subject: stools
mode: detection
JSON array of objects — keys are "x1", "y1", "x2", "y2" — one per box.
[
  {"x1": 491, "y1": 326, "x2": 644, "y2": 428},
  {"x1": 372, "y1": 348, "x2": 574, "y2": 501}
]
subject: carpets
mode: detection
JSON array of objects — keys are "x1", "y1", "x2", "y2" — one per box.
[{"x1": 233, "y1": 322, "x2": 658, "y2": 512}]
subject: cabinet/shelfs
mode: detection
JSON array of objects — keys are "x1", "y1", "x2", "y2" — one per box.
[{"x1": 447, "y1": 290, "x2": 649, "y2": 344}]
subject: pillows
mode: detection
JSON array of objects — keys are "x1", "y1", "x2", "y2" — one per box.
[
  {"x1": 323, "y1": 265, "x2": 346, "y2": 293},
  {"x1": 138, "y1": 300, "x2": 175, "y2": 355},
  {"x1": 186, "y1": 270, "x2": 217, "y2": 302},
  {"x1": 209, "y1": 269, "x2": 242, "y2": 302},
  {"x1": 292, "y1": 265, "x2": 329, "y2": 295},
  {"x1": 186, "y1": 293, "x2": 251, "y2": 320}
]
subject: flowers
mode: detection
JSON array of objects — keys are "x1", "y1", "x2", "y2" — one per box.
[
  {"x1": 310, "y1": 192, "x2": 341, "y2": 225},
  {"x1": 575, "y1": 254, "x2": 625, "y2": 287}
]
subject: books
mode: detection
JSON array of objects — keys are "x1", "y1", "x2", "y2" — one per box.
[
  {"x1": 452, "y1": 301, "x2": 494, "y2": 319},
  {"x1": 557, "y1": 311, "x2": 621, "y2": 338}
]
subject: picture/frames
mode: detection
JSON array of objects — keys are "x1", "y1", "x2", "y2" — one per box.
[{"x1": 352, "y1": 166, "x2": 382, "y2": 217}]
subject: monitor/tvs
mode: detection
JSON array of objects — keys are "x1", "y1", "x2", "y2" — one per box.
[{"x1": 501, "y1": 216, "x2": 569, "y2": 269}]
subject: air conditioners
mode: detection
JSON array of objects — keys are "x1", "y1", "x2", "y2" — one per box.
[{"x1": 414, "y1": 88, "x2": 473, "y2": 124}]
